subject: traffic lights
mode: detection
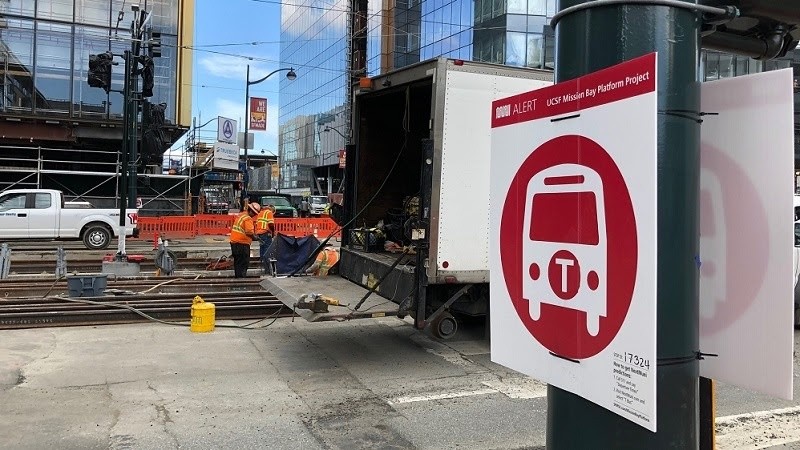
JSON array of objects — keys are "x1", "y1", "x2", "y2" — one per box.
[{"x1": 147, "y1": 25, "x2": 161, "y2": 58}]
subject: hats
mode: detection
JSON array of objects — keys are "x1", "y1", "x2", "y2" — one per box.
[{"x1": 247, "y1": 202, "x2": 260, "y2": 215}]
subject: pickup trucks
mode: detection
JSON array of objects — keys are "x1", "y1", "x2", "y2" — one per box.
[{"x1": 0, "y1": 188, "x2": 140, "y2": 251}]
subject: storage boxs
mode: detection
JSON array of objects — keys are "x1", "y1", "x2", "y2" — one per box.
[
  {"x1": 66, "y1": 274, "x2": 106, "y2": 298},
  {"x1": 349, "y1": 209, "x2": 407, "y2": 253}
]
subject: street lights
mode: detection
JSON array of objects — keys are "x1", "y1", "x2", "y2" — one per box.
[
  {"x1": 261, "y1": 149, "x2": 281, "y2": 196},
  {"x1": 240, "y1": 64, "x2": 297, "y2": 213}
]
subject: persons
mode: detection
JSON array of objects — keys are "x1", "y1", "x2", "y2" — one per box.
[
  {"x1": 255, "y1": 204, "x2": 275, "y2": 274},
  {"x1": 230, "y1": 202, "x2": 261, "y2": 279},
  {"x1": 305, "y1": 239, "x2": 340, "y2": 276}
]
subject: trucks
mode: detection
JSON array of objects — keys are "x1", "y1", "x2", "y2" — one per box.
[{"x1": 258, "y1": 55, "x2": 555, "y2": 340}]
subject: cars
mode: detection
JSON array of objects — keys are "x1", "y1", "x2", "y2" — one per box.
[
  {"x1": 259, "y1": 196, "x2": 299, "y2": 218},
  {"x1": 200, "y1": 188, "x2": 229, "y2": 215},
  {"x1": 306, "y1": 194, "x2": 332, "y2": 218}
]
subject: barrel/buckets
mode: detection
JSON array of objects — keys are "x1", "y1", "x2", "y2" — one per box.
[{"x1": 191, "y1": 296, "x2": 215, "y2": 332}]
